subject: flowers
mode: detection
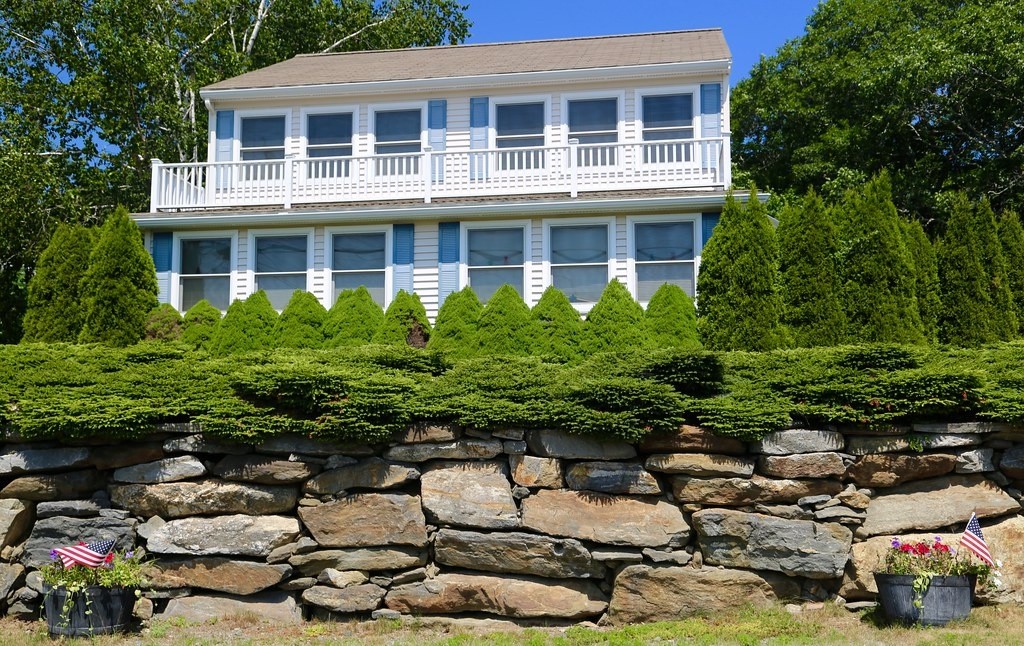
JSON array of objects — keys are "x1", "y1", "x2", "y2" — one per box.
[
  {"x1": 884, "y1": 535, "x2": 1002, "y2": 610},
  {"x1": 39, "y1": 537, "x2": 159, "y2": 646}
]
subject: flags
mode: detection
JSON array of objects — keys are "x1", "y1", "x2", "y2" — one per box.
[
  {"x1": 54, "y1": 540, "x2": 113, "y2": 569},
  {"x1": 958, "y1": 515, "x2": 994, "y2": 565}
]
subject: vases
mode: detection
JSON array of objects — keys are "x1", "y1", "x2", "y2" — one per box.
[
  {"x1": 41, "y1": 580, "x2": 141, "y2": 636},
  {"x1": 871, "y1": 572, "x2": 978, "y2": 629}
]
